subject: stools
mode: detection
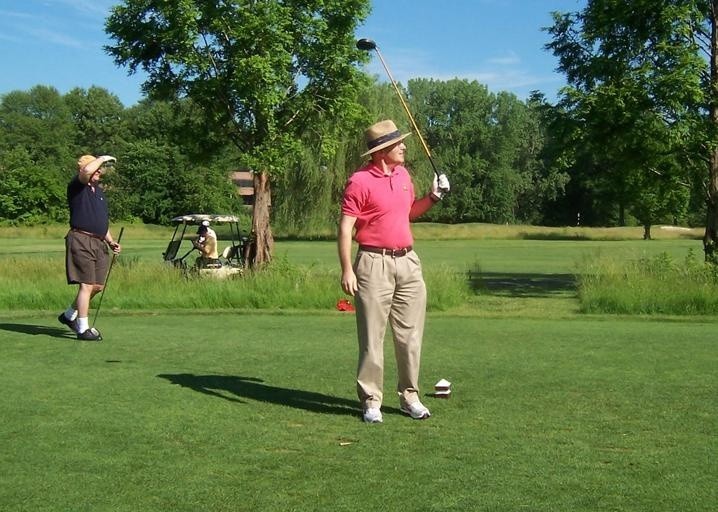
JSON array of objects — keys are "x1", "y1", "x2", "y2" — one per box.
[{"x1": 359, "y1": 244, "x2": 412, "y2": 257}]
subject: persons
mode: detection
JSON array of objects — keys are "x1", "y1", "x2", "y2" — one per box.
[
  {"x1": 57, "y1": 154, "x2": 122, "y2": 341},
  {"x1": 337, "y1": 120, "x2": 455, "y2": 426},
  {"x1": 190, "y1": 225, "x2": 219, "y2": 270},
  {"x1": 199, "y1": 221, "x2": 218, "y2": 257}
]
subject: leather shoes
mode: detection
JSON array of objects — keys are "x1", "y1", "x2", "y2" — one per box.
[
  {"x1": 99, "y1": 155, "x2": 117, "y2": 163},
  {"x1": 429, "y1": 172, "x2": 450, "y2": 202}
]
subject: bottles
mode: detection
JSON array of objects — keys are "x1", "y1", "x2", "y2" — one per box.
[
  {"x1": 78, "y1": 155, "x2": 98, "y2": 172},
  {"x1": 359, "y1": 120, "x2": 412, "y2": 158}
]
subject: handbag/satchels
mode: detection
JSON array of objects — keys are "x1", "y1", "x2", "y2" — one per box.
[{"x1": 426, "y1": 191, "x2": 442, "y2": 205}]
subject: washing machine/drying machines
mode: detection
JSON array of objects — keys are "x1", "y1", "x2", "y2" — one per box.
[
  {"x1": 91, "y1": 226, "x2": 124, "y2": 338},
  {"x1": 356, "y1": 38, "x2": 448, "y2": 194}
]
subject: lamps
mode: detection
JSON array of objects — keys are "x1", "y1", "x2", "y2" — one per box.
[{"x1": 197, "y1": 241, "x2": 236, "y2": 278}]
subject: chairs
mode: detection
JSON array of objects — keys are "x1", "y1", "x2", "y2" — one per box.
[
  {"x1": 77, "y1": 329, "x2": 103, "y2": 340},
  {"x1": 363, "y1": 408, "x2": 384, "y2": 423},
  {"x1": 400, "y1": 400, "x2": 431, "y2": 419},
  {"x1": 58, "y1": 312, "x2": 80, "y2": 332}
]
